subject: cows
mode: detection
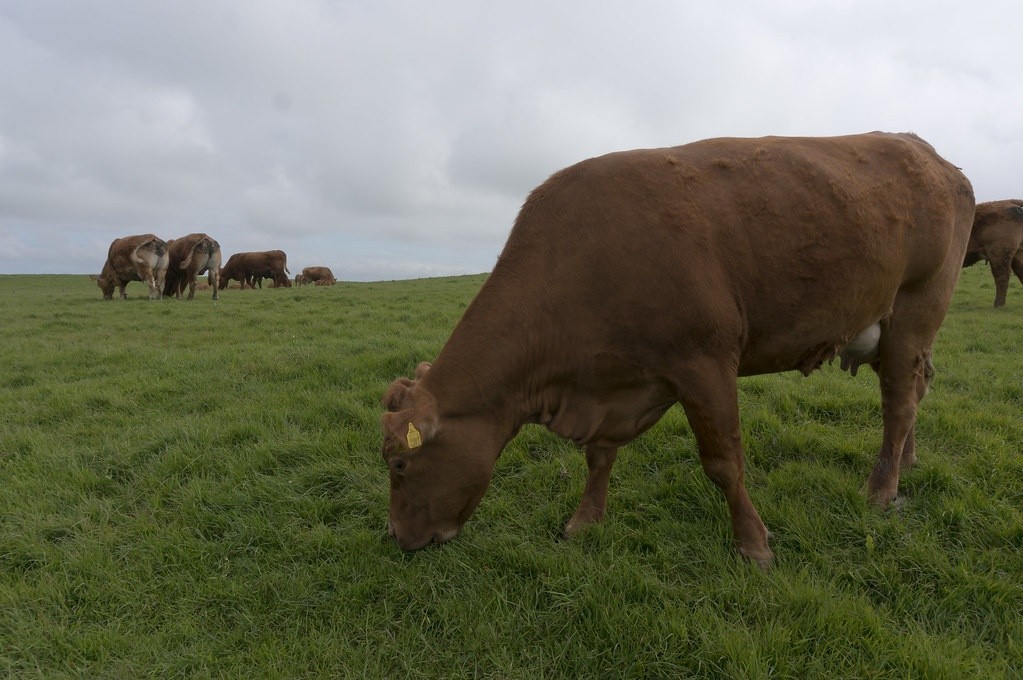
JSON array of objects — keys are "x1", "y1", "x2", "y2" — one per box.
[
  {"x1": 380, "y1": 133, "x2": 976, "y2": 575},
  {"x1": 295, "y1": 265, "x2": 337, "y2": 286},
  {"x1": 194, "y1": 249, "x2": 292, "y2": 291},
  {"x1": 963, "y1": 198, "x2": 1023, "y2": 307},
  {"x1": 89, "y1": 235, "x2": 222, "y2": 301}
]
